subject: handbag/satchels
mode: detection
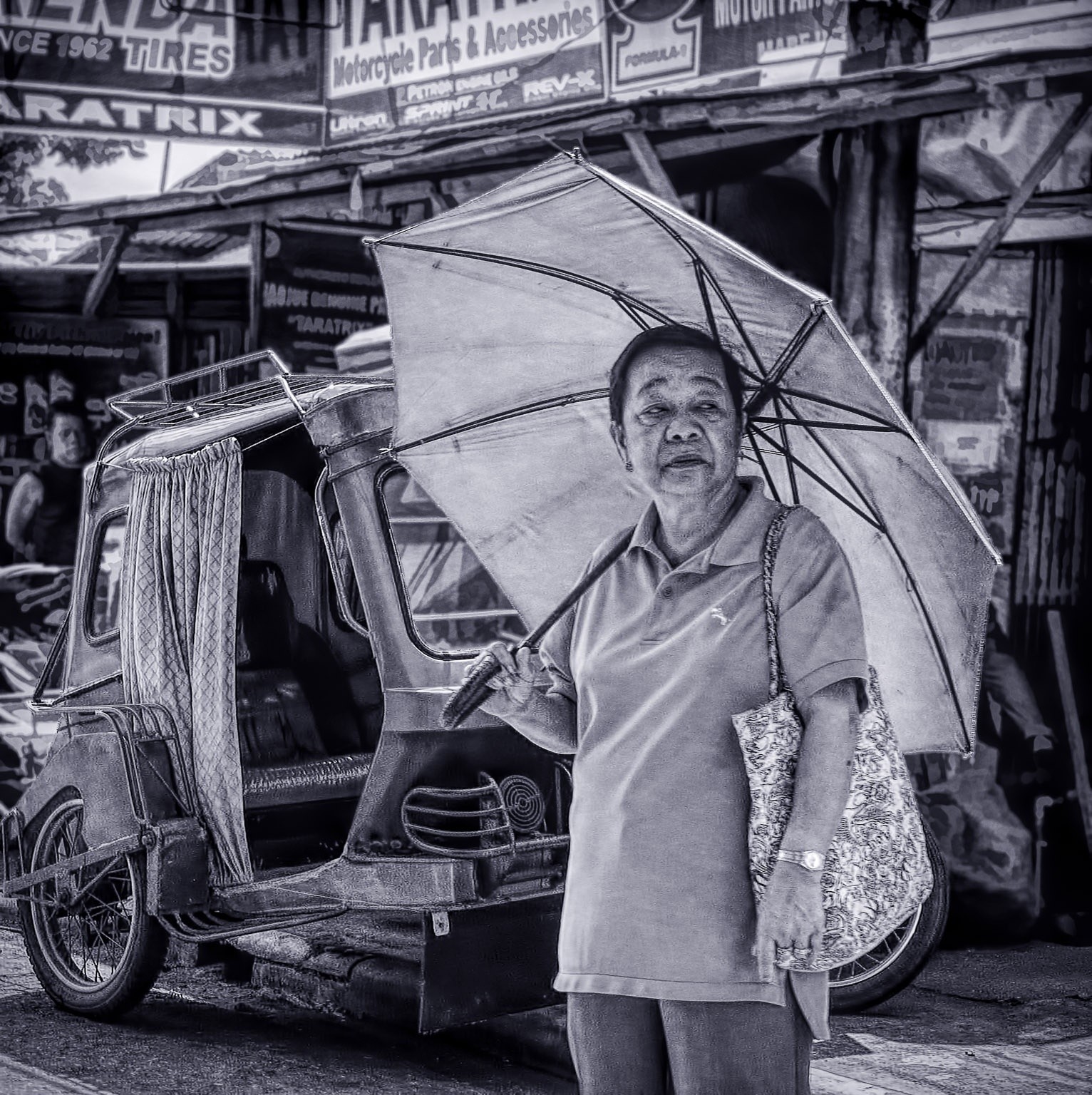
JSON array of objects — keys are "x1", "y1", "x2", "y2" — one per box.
[{"x1": 732, "y1": 505, "x2": 933, "y2": 973}]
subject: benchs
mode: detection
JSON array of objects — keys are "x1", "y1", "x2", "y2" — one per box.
[{"x1": 235, "y1": 665, "x2": 377, "y2": 813}]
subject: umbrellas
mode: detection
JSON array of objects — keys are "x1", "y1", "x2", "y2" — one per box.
[{"x1": 359, "y1": 143, "x2": 1007, "y2": 798}]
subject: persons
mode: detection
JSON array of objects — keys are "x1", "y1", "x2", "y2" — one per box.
[
  {"x1": 5, "y1": 398, "x2": 93, "y2": 567},
  {"x1": 458, "y1": 321, "x2": 870, "y2": 1095},
  {"x1": 234, "y1": 534, "x2": 369, "y2": 757}
]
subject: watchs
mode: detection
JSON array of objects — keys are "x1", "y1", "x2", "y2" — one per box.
[{"x1": 776, "y1": 849, "x2": 825, "y2": 873}]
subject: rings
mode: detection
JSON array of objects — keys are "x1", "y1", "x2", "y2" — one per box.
[
  {"x1": 795, "y1": 948, "x2": 810, "y2": 956},
  {"x1": 777, "y1": 946, "x2": 792, "y2": 956}
]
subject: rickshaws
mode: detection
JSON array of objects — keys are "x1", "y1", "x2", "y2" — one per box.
[{"x1": 0, "y1": 327, "x2": 950, "y2": 1038}]
